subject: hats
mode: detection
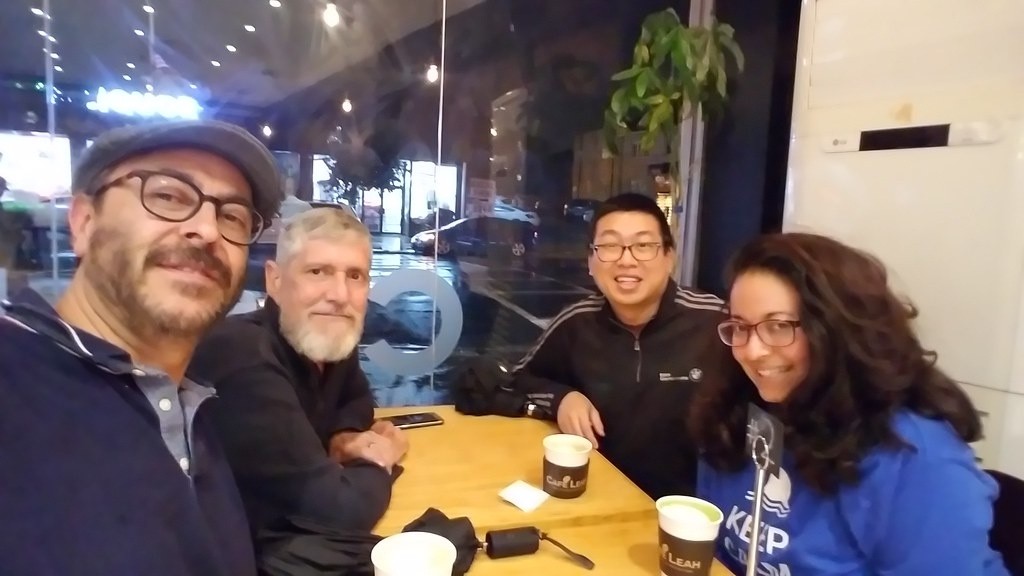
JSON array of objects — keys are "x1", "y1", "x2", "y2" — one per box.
[{"x1": 71, "y1": 118, "x2": 282, "y2": 234}]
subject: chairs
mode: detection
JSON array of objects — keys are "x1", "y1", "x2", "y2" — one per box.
[{"x1": 979, "y1": 467, "x2": 1024, "y2": 576}]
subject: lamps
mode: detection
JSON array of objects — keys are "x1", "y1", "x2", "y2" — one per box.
[
  {"x1": 426, "y1": 59, "x2": 439, "y2": 82},
  {"x1": 323, "y1": 0, "x2": 339, "y2": 27},
  {"x1": 262, "y1": 121, "x2": 272, "y2": 136},
  {"x1": 341, "y1": 93, "x2": 353, "y2": 112}
]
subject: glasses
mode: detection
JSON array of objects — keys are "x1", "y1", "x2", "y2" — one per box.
[
  {"x1": 717, "y1": 317, "x2": 803, "y2": 347},
  {"x1": 95, "y1": 169, "x2": 274, "y2": 248},
  {"x1": 588, "y1": 240, "x2": 665, "y2": 262}
]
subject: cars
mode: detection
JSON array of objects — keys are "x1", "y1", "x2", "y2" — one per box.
[
  {"x1": 406, "y1": 189, "x2": 602, "y2": 263},
  {"x1": 26, "y1": 193, "x2": 80, "y2": 229}
]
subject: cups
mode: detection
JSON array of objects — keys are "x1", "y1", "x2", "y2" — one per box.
[
  {"x1": 542, "y1": 433, "x2": 592, "y2": 500},
  {"x1": 655, "y1": 494, "x2": 724, "y2": 576},
  {"x1": 369, "y1": 530, "x2": 458, "y2": 576}
]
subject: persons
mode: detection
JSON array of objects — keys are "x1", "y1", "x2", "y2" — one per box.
[
  {"x1": 184, "y1": 206, "x2": 406, "y2": 537},
  {"x1": 684, "y1": 232, "x2": 1013, "y2": 576},
  {"x1": 2, "y1": 121, "x2": 285, "y2": 576},
  {"x1": 511, "y1": 192, "x2": 728, "y2": 501}
]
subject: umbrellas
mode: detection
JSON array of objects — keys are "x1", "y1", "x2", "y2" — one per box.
[
  {"x1": 455, "y1": 359, "x2": 549, "y2": 421},
  {"x1": 259, "y1": 507, "x2": 540, "y2": 576}
]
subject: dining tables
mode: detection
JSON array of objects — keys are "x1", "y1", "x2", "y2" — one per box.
[{"x1": 368, "y1": 405, "x2": 740, "y2": 576}]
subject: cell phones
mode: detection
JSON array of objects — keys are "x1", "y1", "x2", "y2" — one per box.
[{"x1": 374, "y1": 412, "x2": 444, "y2": 429}]
